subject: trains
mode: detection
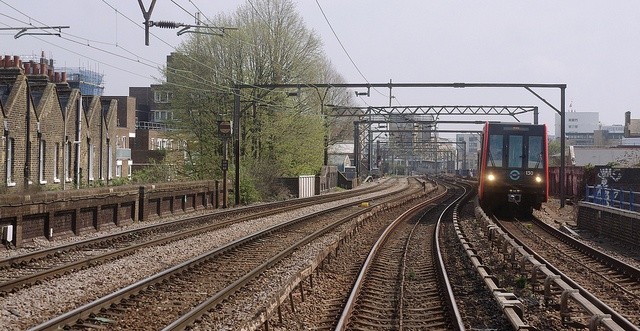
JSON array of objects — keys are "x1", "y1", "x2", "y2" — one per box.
[{"x1": 478, "y1": 120, "x2": 550, "y2": 214}]
